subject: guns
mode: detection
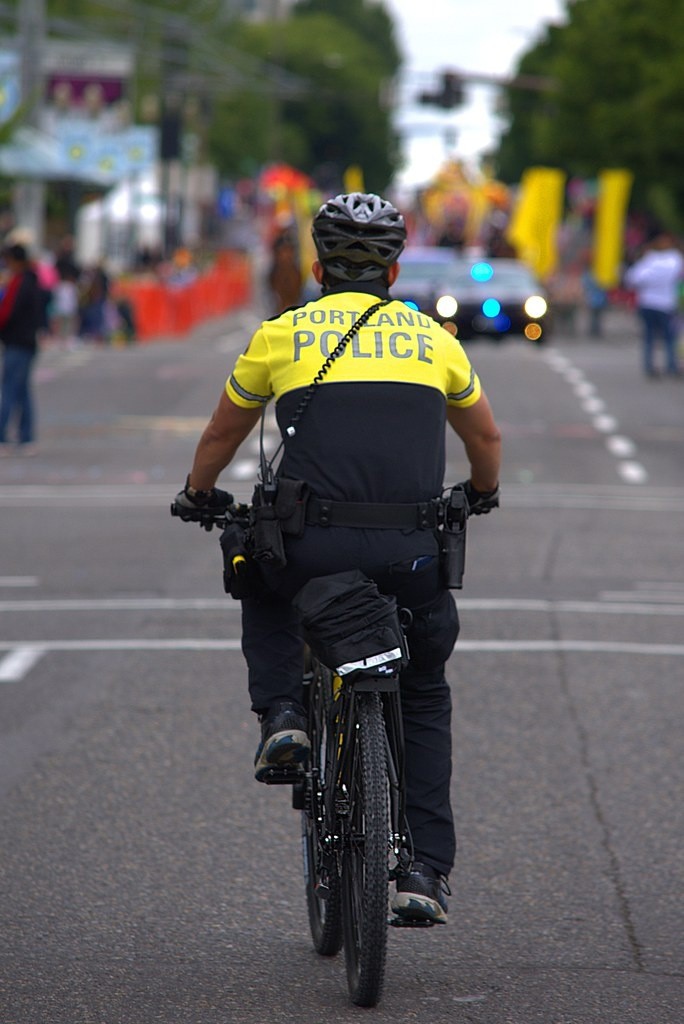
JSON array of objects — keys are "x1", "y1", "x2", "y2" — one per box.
[{"x1": 448, "y1": 490, "x2": 465, "y2": 589}]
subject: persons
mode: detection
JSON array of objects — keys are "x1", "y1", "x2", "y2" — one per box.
[
  {"x1": 174, "y1": 191, "x2": 501, "y2": 923},
  {"x1": 0, "y1": 244, "x2": 51, "y2": 442},
  {"x1": 624, "y1": 234, "x2": 684, "y2": 378}
]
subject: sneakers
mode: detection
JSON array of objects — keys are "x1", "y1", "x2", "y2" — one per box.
[
  {"x1": 253, "y1": 709, "x2": 314, "y2": 784},
  {"x1": 390, "y1": 873, "x2": 447, "y2": 924}
]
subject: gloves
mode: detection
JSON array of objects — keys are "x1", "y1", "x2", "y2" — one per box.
[
  {"x1": 173, "y1": 475, "x2": 235, "y2": 532},
  {"x1": 460, "y1": 479, "x2": 502, "y2": 515}
]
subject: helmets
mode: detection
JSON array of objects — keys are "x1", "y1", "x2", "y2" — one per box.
[{"x1": 309, "y1": 190, "x2": 407, "y2": 283}]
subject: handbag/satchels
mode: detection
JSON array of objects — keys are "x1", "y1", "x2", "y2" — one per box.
[{"x1": 293, "y1": 568, "x2": 410, "y2": 682}]
